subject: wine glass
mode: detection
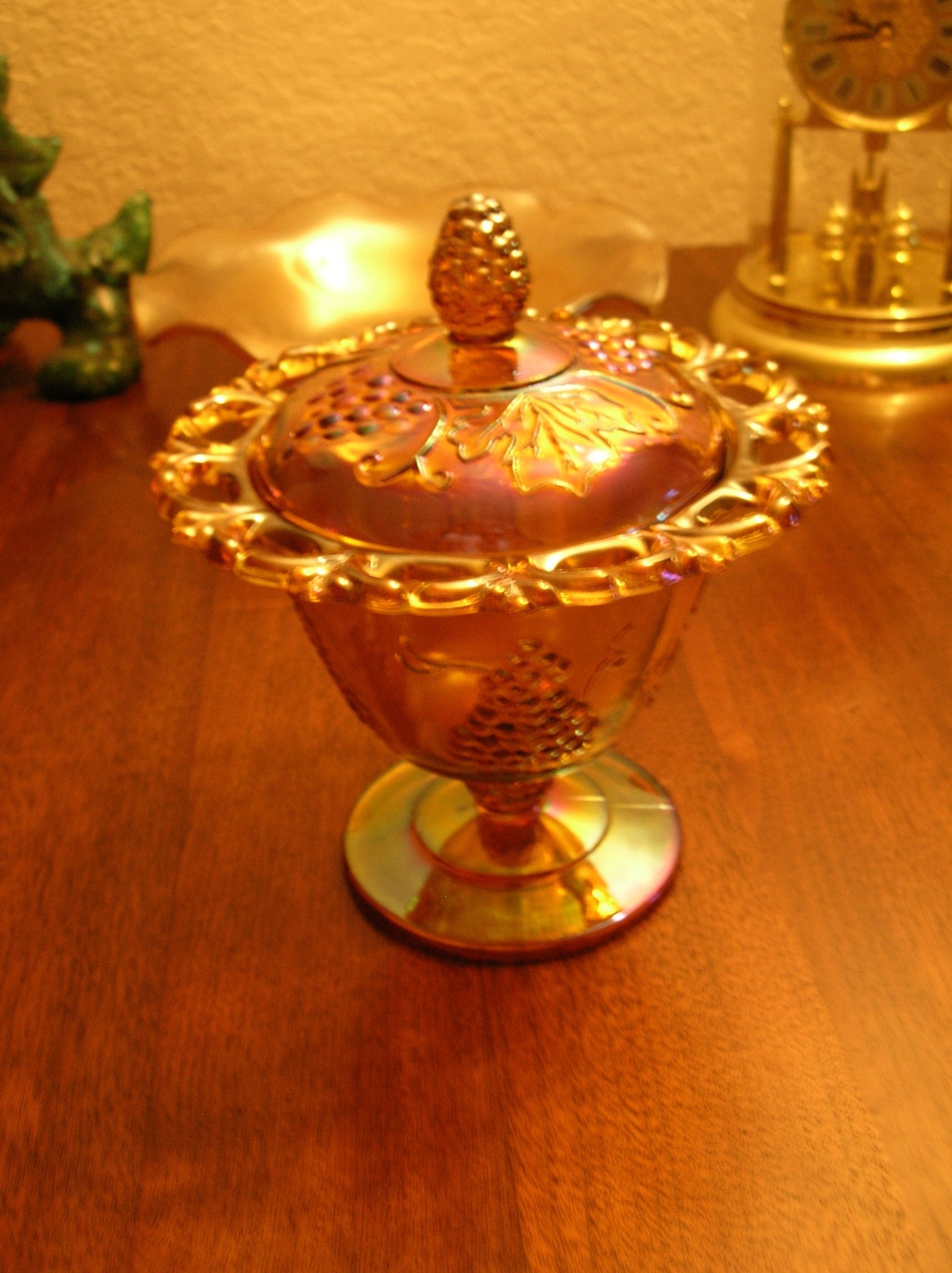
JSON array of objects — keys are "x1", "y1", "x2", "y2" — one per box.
[{"x1": 154, "y1": 193, "x2": 828, "y2": 961}]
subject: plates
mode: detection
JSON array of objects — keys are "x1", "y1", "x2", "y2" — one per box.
[{"x1": 130, "y1": 182, "x2": 670, "y2": 361}]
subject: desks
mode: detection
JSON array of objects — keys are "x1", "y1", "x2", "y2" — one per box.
[{"x1": 0, "y1": 242, "x2": 952, "y2": 1273}]
